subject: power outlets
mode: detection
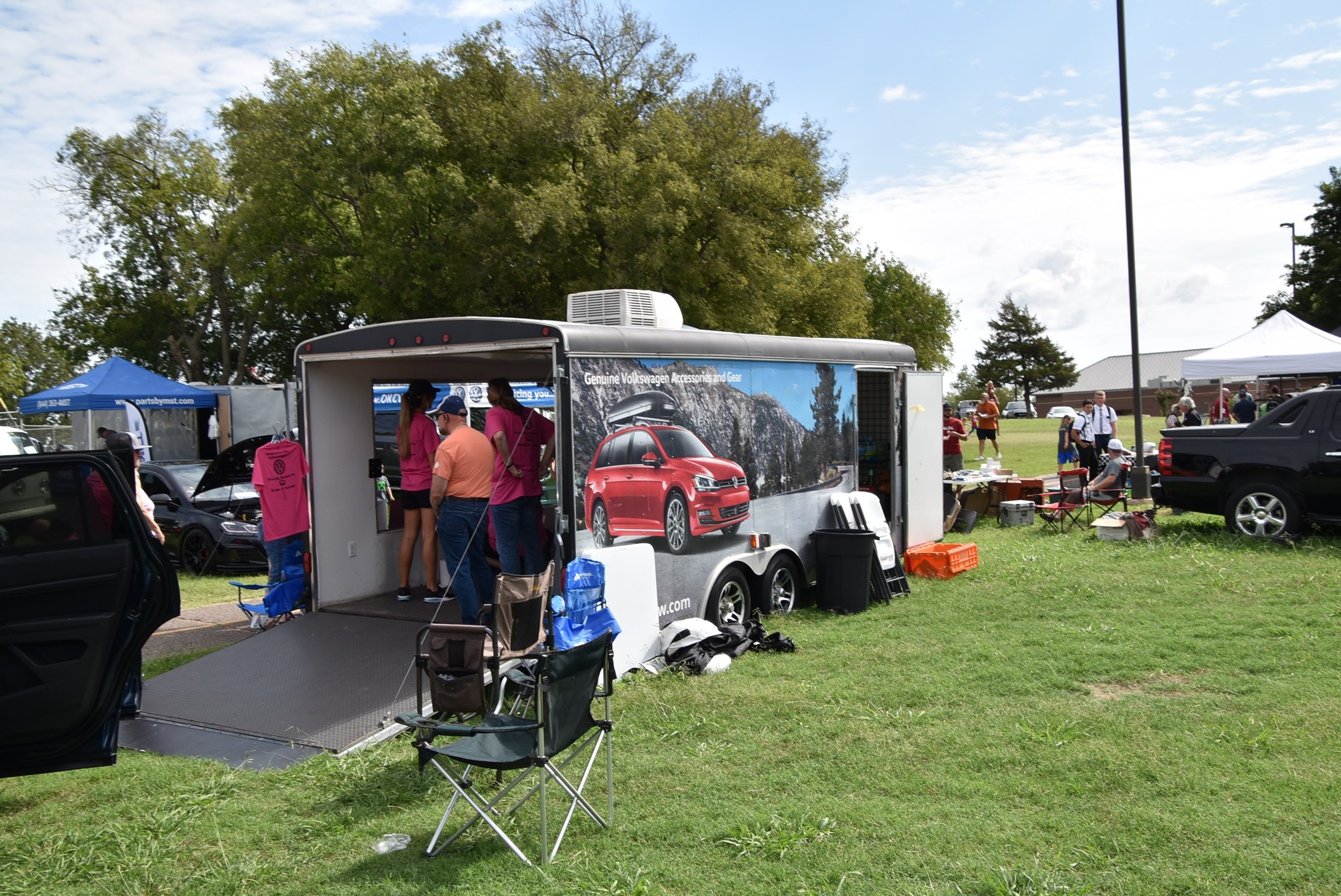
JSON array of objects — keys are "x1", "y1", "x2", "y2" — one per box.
[{"x1": 349, "y1": 541, "x2": 357, "y2": 557}]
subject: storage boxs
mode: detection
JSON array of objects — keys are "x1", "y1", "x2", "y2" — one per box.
[
  {"x1": 999, "y1": 500, "x2": 1035, "y2": 527},
  {"x1": 1090, "y1": 512, "x2": 1130, "y2": 541},
  {"x1": 904, "y1": 541, "x2": 978, "y2": 580}
]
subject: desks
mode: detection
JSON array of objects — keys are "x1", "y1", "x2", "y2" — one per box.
[
  {"x1": 1097, "y1": 450, "x2": 1158, "y2": 478},
  {"x1": 943, "y1": 474, "x2": 1018, "y2": 532}
]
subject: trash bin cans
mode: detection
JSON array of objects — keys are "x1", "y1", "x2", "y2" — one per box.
[{"x1": 809, "y1": 528, "x2": 880, "y2": 617}]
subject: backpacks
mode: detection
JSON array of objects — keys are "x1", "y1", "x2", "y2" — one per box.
[{"x1": 1069, "y1": 414, "x2": 1086, "y2": 442}]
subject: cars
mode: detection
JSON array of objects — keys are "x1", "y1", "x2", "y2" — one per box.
[
  {"x1": 0, "y1": 449, "x2": 181, "y2": 782},
  {"x1": 0, "y1": 426, "x2": 45, "y2": 456},
  {"x1": 585, "y1": 390, "x2": 752, "y2": 556},
  {"x1": 954, "y1": 400, "x2": 984, "y2": 421},
  {"x1": 1046, "y1": 406, "x2": 1079, "y2": 419},
  {"x1": 1151, "y1": 385, "x2": 1341, "y2": 549},
  {"x1": 138, "y1": 427, "x2": 307, "y2": 577},
  {"x1": 1002, "y1": 401, "x2": 1038, "y2": 419}
]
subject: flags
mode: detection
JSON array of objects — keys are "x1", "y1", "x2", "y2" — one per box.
[{"x1": 124, "y1": 399, "x2": 154, "y2": 462}]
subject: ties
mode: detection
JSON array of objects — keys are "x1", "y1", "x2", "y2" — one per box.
[{"x1": 1099, "y1": 408, "x2": 1103, "y2": 433}]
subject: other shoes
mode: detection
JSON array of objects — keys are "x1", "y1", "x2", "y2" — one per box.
[{"x1": 1039, "y1": 512, "x2": 1059, "y2": 523}]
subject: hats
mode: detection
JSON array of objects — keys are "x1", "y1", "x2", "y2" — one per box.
[
  {"x1": 408, "y1": 379, "x2": 441, "y2": 400},
  {"x1": 105, "y1": 432, "x2": 153, "y2": 450},
  {"x1": 1107, "y1": 438, "x2": 1123, "y2": 456},
  {"x1": 96, "y1": 426, "x2": 105, "y2": 439},
  {"x1": 424, "y1": 395, "x2": 468, "y2": 416},
  {"x1": 943, "y1": 403, "x2": 953, "y2": 410}
]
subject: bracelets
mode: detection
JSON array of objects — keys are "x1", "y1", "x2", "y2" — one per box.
[{"x1": 505, "y1": 461, "x2": 513, "y2": 468}]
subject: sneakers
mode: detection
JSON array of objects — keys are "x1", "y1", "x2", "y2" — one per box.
[
  {"x1": 423, "y1": 584, "x2": 456, "y2": 602},
  {"x1": 394, "y1": 585, "x2": 414, "y2": 601},
  {"x1": 996, "y1": 452, "x2": 1001, "y2": 459},
  {"x1": 974, "y1": 455, "x2": 984, "y2": 461}
]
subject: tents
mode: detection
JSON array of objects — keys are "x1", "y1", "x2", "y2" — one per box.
[
  {"x1": 19, "y1": 356, "x2": 220, "y2": 456},
  {"x1": 1180, "y1": 310, "x2": 1341, "y2": 423}
]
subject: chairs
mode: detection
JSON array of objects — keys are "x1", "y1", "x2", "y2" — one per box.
[
  {"x1": 393, "y1": 558, "x2": 622, "y2": 869},
  {"x1": 1085, "y1": 461, "x2": 1133, "y2": 530},
  {"x1": 829, "y1": 491, "x2": 911, "y2": 605},
  {"x1": 1026, "y1": 468, "x2": 1090, "y2": 535},
  {"x1": 227, "y1": 539, "x2": 306, "y2": 633}
]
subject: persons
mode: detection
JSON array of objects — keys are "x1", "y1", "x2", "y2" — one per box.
[
  {"x1": 376, "y1": 463, "x2": 395, "y2": 506},
  {"x1": 1209, "y1": 383, "x2": 1293, "y2": 425},
  {"x1": 1071, "y1": 398, "x2": 1099, "y2": 496},
  {"x1": 427, "y1": 395, "x2": 497, "y2": 625},
  {"x1": 87, "y1": 431, "x2": 165, "y2": 545},
  {"x1": 943, "y1": 402, "x2": 969, "y2": 500},
  {"x1": 1039, "y1": 439, "x2": 1128, "y2": 522},
  {"x1": 397, "y1": 378, "x2": 457, "y2": 602},
  {"x1": 97, "y1": 427, "x2": 118, "y2": 439},
  {"x1": 973, "y1": 392, "x2": 1002, "y2": 460},
  {"x1": 1164, "y1": 403, "x2": 1182, "y2": 429},
  {"x1": 483, "y1": 377, "x2": 554, "y2": 574},
  {"x1": 1178, "y1": 396, "x2": 1203, "y2": 426},
  {"x1": 962, "y1": 380, "x2": 1003, "y2": 438},
  {"x1": 1057, "y1": 415, "x2": 1080, "y2": 477},
  {"x1": 1088, "y1": 391, "x2": 1118, "y2": 455},
  {"x1": 256, "y1": 519, "x2": 290, "y2": 594}
]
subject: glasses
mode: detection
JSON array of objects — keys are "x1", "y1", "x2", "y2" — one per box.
[
  {"x1": 980, "y1": 396, "x2": 988, "y2": 399},
  {"x1": 435, "y1": 413, "x2": 446, "y2": 420}
]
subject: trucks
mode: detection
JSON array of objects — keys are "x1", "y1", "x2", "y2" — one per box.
[{"x1": 112, "y1": 288, "x2": 943, "y2": 776}]
